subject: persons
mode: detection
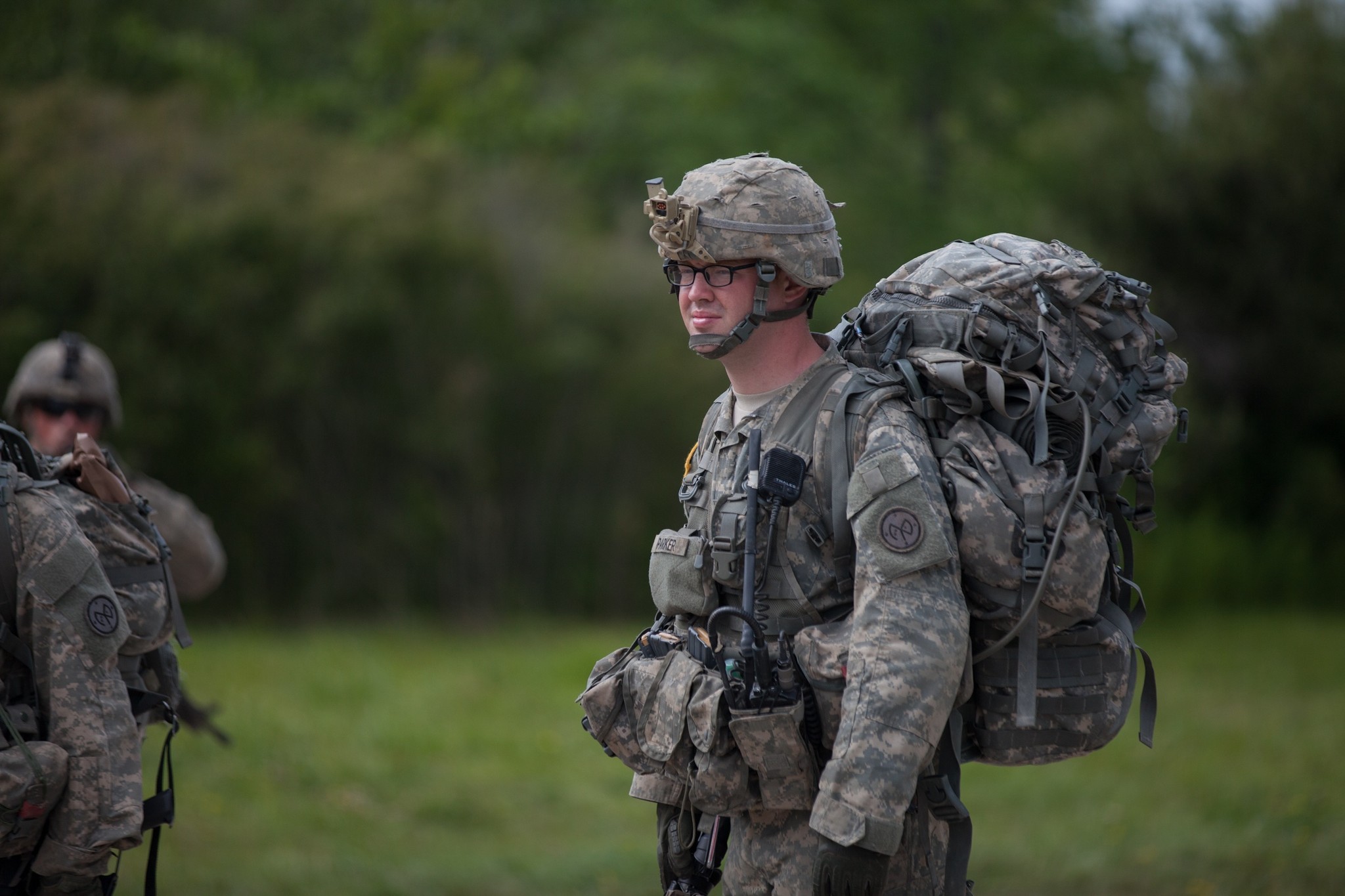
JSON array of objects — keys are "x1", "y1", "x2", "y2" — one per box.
[
  {"x1": 0, "y1": 457, "x2": 144, "y2": 896},
  {"x1": 12, "y1": 336, "x2": 121, "y2": 452},
  {"x1": 576, "y1": 153, "x2": 972, "y2": 896}
]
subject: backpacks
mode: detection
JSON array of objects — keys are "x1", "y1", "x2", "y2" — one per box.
[
  {"x1": 121, "y1": 465, "x2": 230, "y2": 608},
  {"x1": 13, "y1": 428, "x2": 190, "y2": 722},
  {"x1": 824, "y1": 228, "x2": 1192, "y2": 779}
]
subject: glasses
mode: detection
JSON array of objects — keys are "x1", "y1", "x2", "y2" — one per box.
[{"x1": 660, "y1": 256, "x2": 761, "y2": 287}]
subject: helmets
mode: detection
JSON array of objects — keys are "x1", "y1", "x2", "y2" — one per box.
[
  {"x1": 5, "y1": 333, "x2": 126, "y2": 435},
  {"x1": 639, "y1": 152, "x2": 851, "y2": 290}
]
